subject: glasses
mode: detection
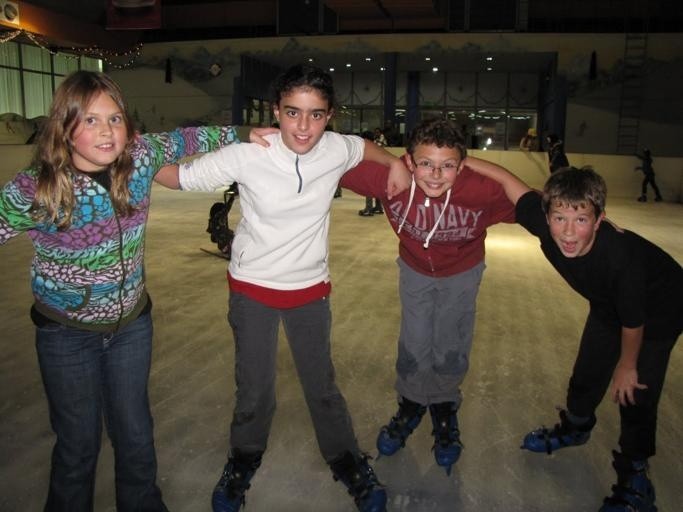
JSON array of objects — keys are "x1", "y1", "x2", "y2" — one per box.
[{"x1": 410, "y1": 157, "x2": 462, "y2": 174}]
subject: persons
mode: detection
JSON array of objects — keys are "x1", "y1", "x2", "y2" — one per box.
[
  {"x1": 459, "y1": 154, "x2": 683, "y2": 512},
  {"x1": 632, "y1": 147, "x2": 662, "y2": 202},
  {"x1": 520, "y1": 128, "x2": 540, "y2": 152},
  {"x1": 0, "y1": 69, "x2": 281, "y2": 512},
  {"x1": 359, "y1": 129, "x2": 387, "y2": 215},
  {"x1": 546, "y1": 133, "x2": 569, "y2": 173},
  {"x1": 336, "y1": 116, "x2": 625, "y2": 466},
  {"x1": 153, "y1": 61, "x2": 413, "y2": 512}
]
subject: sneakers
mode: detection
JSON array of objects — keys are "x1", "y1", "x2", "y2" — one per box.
[
  {"x1": 428, "y1": 401, "x2": 462, "y2": 468},
  {"x1": 327, "y1": 450, "x2": 389, "y2": 511},
  {"x1": 376, "y1": 395, "x2": 428, "y2": 456},
  {"x1": 211, "y1": 449, "x2": 264, "y2": 512},
  {"x1": 523, "y1": 408, "x2": 597, "y2": 454},
  {"x1": 358, "y1": 208, "x2": 373, "y2": 216},
  {"x1": 372, "y1": 206, "x2": 383, "y2": 213},
  {"x1": 594, "y1": 450, "x2": 657, "y2": 512}
]
